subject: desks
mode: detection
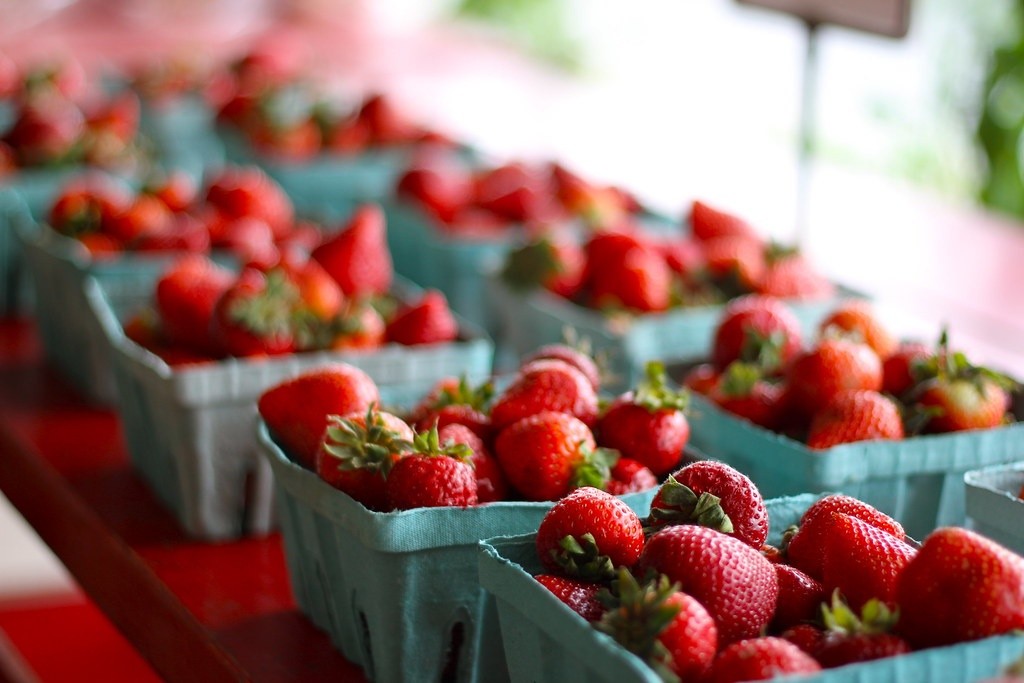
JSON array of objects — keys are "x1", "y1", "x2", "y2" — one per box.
[{"x1": 1, "y1": 311, "x2": 362, "y2": 683}]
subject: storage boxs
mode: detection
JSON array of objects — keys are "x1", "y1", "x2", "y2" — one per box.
[
  {"x1": 0, "y1": 187, "x2": 338, "y2": 416},
  {"x1": 660, "y1": 354, "x2": 1024, "y2": 541},
  {"x1": 210, "y1": 117, "x2": 478, "y2": 226},
  {"x1": 253, "y1": 377, "x2": 715, "y2": 683},
  {"x1": 104, "y1": 70, "x2": 327, "y2": 165},
  {"x1": 475, "y1": 489, "x2": 1024, "y2": 683},
  {"x1": 349, "y1": 191, "x2": 682, "y2": 334},
  {"x1": 963, "y1": 460, "x2": 1024, "y2": 554},
  {"x1": 81, "y1": 269, "x2": 495, "y2": 544},
  {"x1": 474, "y1": 255, "x2": 873, "y2": 397}
]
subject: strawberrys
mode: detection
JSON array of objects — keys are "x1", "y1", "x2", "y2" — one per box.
[{"x1": 2, "y1": 51, "x2": 1024, "y2": 682}]
